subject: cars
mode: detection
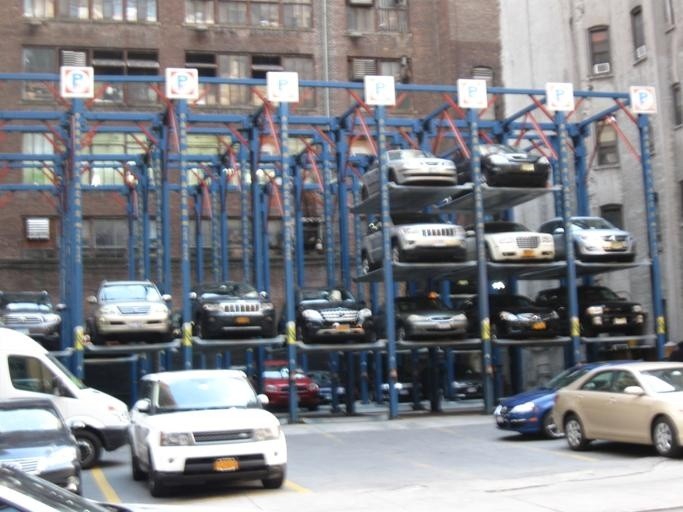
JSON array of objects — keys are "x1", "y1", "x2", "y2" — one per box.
[
  {"x1": 552, "y1": 360, "x2": 682, "y2": 459},
  {"x1": 180, "y1": 280, "x2": 277, "y2": 342},
  {"x1": 0, "y1": 291, "x2": 66, "y2": 348},
  {"x1": 88, "y1": 279, "x2": 176, "y2": 342},
  {"x1": 281, "y1": 286, "x2": 371, "y2": 346},
  {"x1": 493, "y1": 361, "x2": 639, "y2": 439}
]
